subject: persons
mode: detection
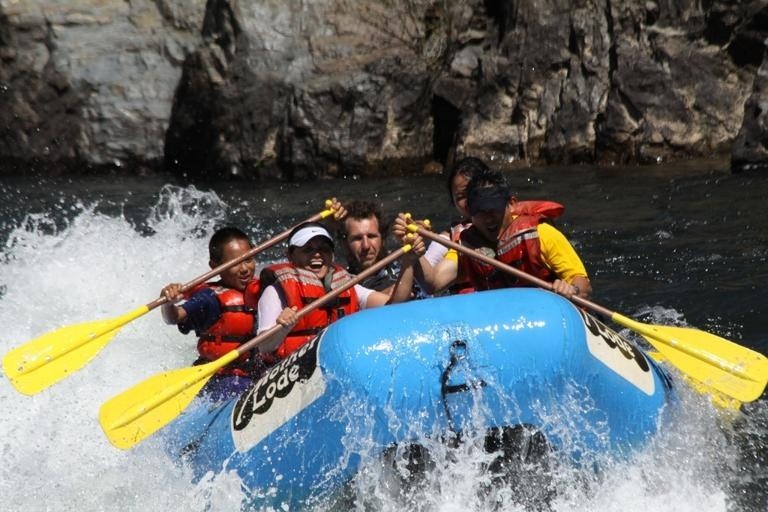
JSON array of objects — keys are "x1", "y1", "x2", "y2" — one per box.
[
  {"x1": 336, "y1": 200, "x2": 444, "y2": 302},
  {"x1": 159, "y1": 227, "x2": 265, "y2": 400},
  {"x1": 391, "y1": 168, "x2": 593, "y2": 299},
  {"x1": 250, "y1": 221, "x2": 428, "y2": 382},
  {"x1": 421, "y1": 155, "x2": 493, "y2": 267}
]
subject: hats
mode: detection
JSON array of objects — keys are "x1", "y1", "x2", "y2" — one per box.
[
  {"x1": 466, "y1": 184, "x2": 511, "y2": 216},
  {"x1": 288, "y1": 225, "x2": 335, "y2": 250}
]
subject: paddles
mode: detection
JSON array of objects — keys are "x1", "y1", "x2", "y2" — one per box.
[
  {"x1": 646, "y1": 351, "x2": 742, "y2": 412},
  {"x1": 2, "y1": 199, "x2": 339, "y2": 395},
  {"x1": 98, "y1": 233, "x2": 416, "y2": 450},
  {"x1": 404, "y1": 213, "x2": 768, "y2": 403}
]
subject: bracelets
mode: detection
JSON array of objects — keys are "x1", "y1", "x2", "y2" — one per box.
[{"x1": 568, "y1": 283, "x2": 579, "y2": 296}]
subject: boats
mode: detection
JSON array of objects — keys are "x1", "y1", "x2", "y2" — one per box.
[{"x1": 161, "y1": 285, "x2": 675, "y2": 512}]
